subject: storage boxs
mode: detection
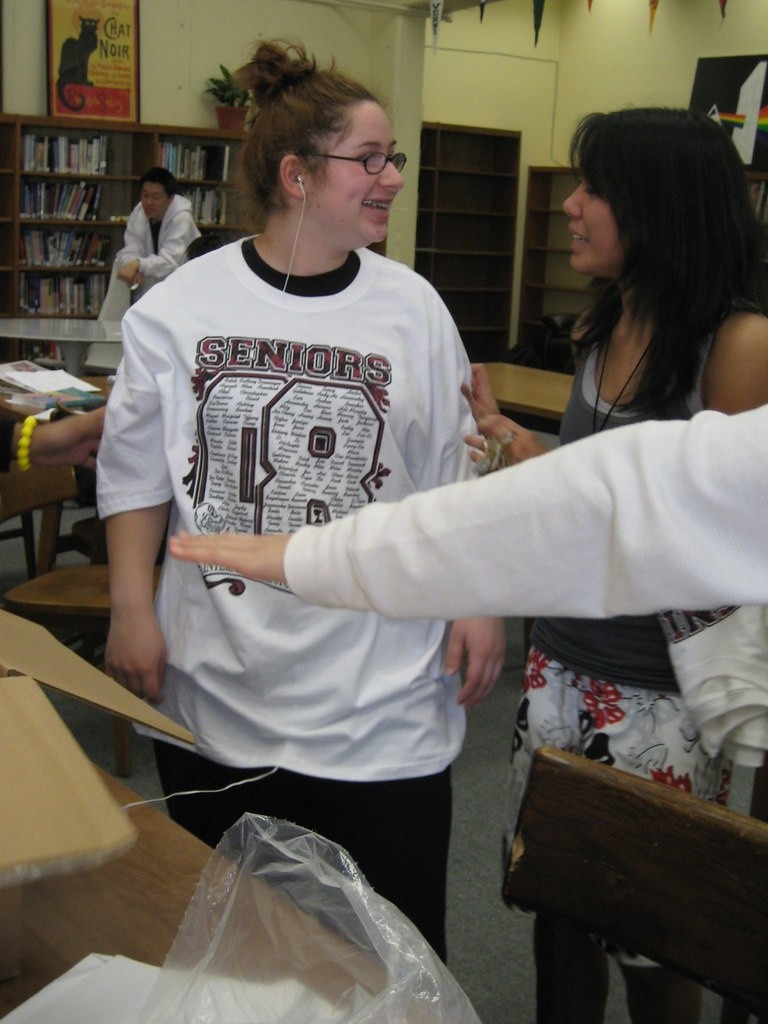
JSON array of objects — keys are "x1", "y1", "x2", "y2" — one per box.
[{"x1": 1, "y1": 610, "x2": 195, "y2": 983}]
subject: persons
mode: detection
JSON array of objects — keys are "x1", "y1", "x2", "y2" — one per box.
[
  {"x1": 114, "y1": 167, "x2": 226, "y2": 304},
  {"x1": 169, "y1": 403, "x2": 768, "y2": 617},
  {"x1": 461, "y1": 108, "x2": 768, "y2": 1023},
  {"x1": 0, "y1": 404, "x2": 107, "y2": 473},
  {"x1": 95, "y1": 41, "x2": 502, "y2": 970}
]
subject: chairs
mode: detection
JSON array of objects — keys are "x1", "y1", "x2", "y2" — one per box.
[
  {"x1": 0, "y1": 464, "x2": 165, "y2": 777},
  {"x1": 503, "y1": 748, "x2": 768, "y2": 1024}
]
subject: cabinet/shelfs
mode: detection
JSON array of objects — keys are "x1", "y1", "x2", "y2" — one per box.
[
  {"x1": 416, "y1": 120, "x2": 521, "y2": 364},
  {"x1": 0, "y1": 113, "x2": 267, "y2": 362},
  {"x1": 517, "y1": 167, "x2": 768, "y2": 347}
]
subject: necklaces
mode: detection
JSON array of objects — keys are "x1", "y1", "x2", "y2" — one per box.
[{"x1": 592, "y1": 318, "x2": 651, "y2": 433}]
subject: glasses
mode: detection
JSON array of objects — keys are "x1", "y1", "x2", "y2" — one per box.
[{"x1": 295, "y1": 149, "x2": 407, "y2": 175}]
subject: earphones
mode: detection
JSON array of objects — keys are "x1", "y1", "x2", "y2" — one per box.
[{"x1": 296, "y1": 174, "x2": 303, "y2": 185}]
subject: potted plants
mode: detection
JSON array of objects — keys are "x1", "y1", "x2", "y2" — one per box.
[{"x1": 204, "y1": 64, "x2": 251, "y2": 133}]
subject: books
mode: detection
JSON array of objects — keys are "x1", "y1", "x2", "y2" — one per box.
[{"x1": 0, "y1": 136, "x2": 222, "y2": 410}]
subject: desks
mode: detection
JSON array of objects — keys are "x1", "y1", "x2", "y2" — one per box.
[
  {"x1": 1, "y1": 765, "x2": 438, "y2": 1024},
  {"x1": 485, "y1": 363, "x2": 576, "y2": 437},
  {"x1": 0, "y1": 319, "x2": 123, "y2": 377},
  {"x1": 1, "y1": 377, "x2": 115, "y2": 575}
]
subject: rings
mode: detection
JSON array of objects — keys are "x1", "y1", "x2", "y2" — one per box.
[{"x1": 504, "y1": 430, "x2": 517, "y2": 448}]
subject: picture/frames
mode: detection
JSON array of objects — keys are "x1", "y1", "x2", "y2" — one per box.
[{"x1": 45, "y1": 0, "x2": 141, "y2": 123}]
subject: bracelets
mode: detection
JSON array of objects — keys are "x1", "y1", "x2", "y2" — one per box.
[{"x1": 16, "y1": 416, "x2": 37, "y2": 472}]
